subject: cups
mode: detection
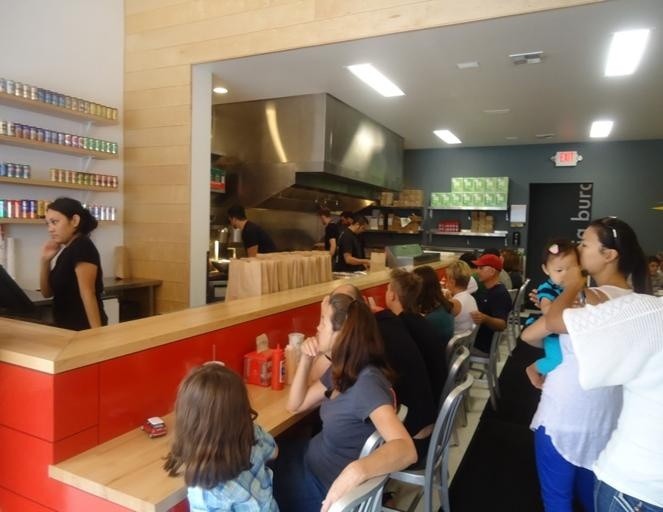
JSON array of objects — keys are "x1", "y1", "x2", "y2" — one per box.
[
  {"x1": 0, "y1": 236, "x2": 16, "y2": 281},
  {"x1": 231, "y1": 227, "x2": 241, "y2": 242},
  {"x1": 214, "y1": 231, "x2": 230, "y2": 245}
]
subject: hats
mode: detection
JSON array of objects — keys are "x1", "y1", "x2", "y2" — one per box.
[{"x1": 471, "y1": 254, "x2": 502, "y2": 271}]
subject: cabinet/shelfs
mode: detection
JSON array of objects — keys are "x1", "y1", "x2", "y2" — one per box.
[
  {"x1": 425, "y1": 206, "x2": 509, "y2": 247},
  {"x1": 0, "y1": 91, "x2": 120, "y2": 225},
  {"x1": 364, "y1": 206, "x2": 421, "y2": 235}
]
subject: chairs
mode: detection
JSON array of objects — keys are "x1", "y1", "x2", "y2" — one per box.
[{"x1": 324, "y1": 274, "x2": 533, "y2": 511}]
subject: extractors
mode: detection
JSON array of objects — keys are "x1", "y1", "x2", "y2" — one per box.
[{"x1": 213, "y1": 91, "x2": 405, "y2": 215}]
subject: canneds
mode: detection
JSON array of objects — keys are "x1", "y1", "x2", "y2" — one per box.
[
  {"x1": 0, "y1": 120, "x2": 118, "y2": 154},
  {"x1": 51, "y1": 168, "x2": 119, "y2": 187},
  {"x1": 82, "y1": 204, "x2": 116, "y2": 222},
  {"x1": 0, "y1": 199, "x2": 53, "y2": 220},
  {"x1": 0, "y1": 163, "x2": 31, "y2": 178},
  {"x1": 0, "y1": 77, "x2": 118, "y2": 121}
]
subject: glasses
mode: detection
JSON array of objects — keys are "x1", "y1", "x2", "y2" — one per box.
[{"x1": 602, "y1": 215, "x2": 623, "y2": 251}]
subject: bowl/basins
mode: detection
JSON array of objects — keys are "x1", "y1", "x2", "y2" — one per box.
[{"x1": 211, "y1": 261, "x2": 228, "y2": 273}]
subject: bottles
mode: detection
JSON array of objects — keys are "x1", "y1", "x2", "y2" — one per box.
[
  {"x1": 441, "y1": 275, "x2": 447, "y2": 289},
  {"x1": 438, "y1": 220, "x2": 461, "y2": 232},
  {"x1": 284, "y1": 341, "x2": 296, "y2": 385}
]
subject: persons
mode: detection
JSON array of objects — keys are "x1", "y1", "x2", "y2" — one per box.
[
  {"x1": 227, "y1": 205, "x2": 275, "y2": 257},
  {"x1": 0, "y1": 266, "x2": 42, "y2": 322},
  {"x1": 160, "y1": 363, "x2": 278, "y2": 511},
  {"x1": 40, "y1": 197, "x2": 107, "y2": 330}
]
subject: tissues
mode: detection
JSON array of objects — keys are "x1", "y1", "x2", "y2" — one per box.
[{"x1": 243, "y1": 333, "x2": 275, "y2": 388}]
